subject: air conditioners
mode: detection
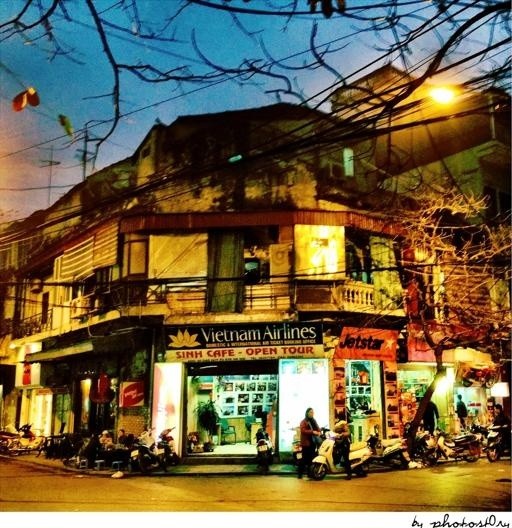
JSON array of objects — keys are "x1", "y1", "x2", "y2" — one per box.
[{"x1": 70, "y1": 297, "x2": 86, "y2": 318}]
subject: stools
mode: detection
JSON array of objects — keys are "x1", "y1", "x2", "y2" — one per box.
[{"x1": 79, "y1": 458, "x2": 123, "y2": 471}]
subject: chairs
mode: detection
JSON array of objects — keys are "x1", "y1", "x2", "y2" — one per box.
[{"x1": 218, "y1": 417, "x2": 236, "y2": 442}]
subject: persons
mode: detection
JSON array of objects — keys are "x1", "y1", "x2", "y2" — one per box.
[
  {"x1": 490, "y1": 403, "x2": 512, "y2": 460},
  {"x1": 333, "y1": 413, "x2": 352, "y2": 481},
  {"x1": 422, "y1": 401, "x2": 439, "y2": 436},
  {"x1": 454, "y1": 394, "x2": 468, "y2": 431},
  {"x1": 297, "y1": 407, "x2": 321, "y2": 478},
  {"x1": 76, "y1": 429, "x2": 175, "y2": 472}
]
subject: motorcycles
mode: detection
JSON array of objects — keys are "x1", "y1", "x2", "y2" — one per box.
[
  {"x1": 252, "y1": 425, "x2": 274, "y2": 470},
  {"x1": 0, "y1": 422, "x2": 37, "y2": 455},
  {"x1": 125, "y1": 426, "x2": 180, "y2": 473},
  {"x1": 289, "y1": 420, "x2": 511, "y2": 481}
]
